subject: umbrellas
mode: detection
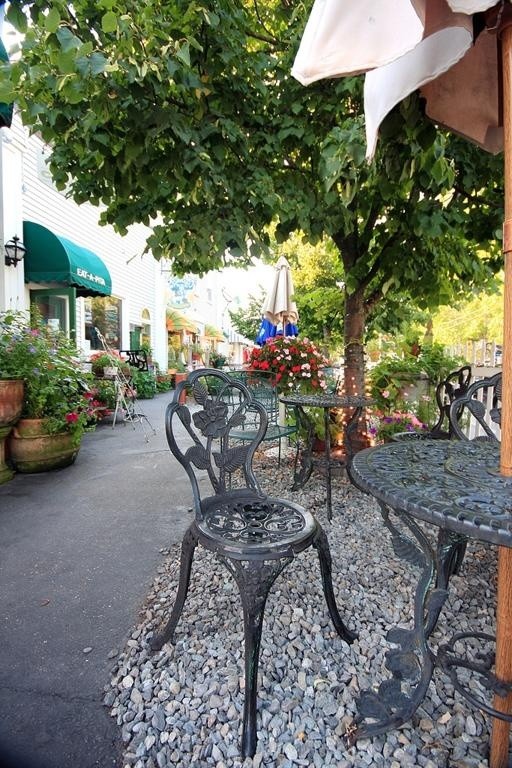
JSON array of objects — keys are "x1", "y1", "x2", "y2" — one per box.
[
  {"x1": 254, "y1": 318, "x2": 298, "y2": 348},
  {"x1": 290, "y1": 0, "x2": 512, "y2": 768},
  {"x1": 252, "y1": 253, "x2": 299, "y2": 337}
]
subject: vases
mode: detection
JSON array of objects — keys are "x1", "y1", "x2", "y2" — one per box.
[{"x1": 0, "y1": 380, "x2": 25, "y2": 484}]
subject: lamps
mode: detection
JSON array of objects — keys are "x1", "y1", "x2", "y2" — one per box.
[{"x1": 5, "y1": 234, "x2": 27, "y2": 268}]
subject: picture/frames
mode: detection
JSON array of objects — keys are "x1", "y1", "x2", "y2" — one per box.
[{"x1": 8, "y1": 419, "x2": 80, "y2": 475}]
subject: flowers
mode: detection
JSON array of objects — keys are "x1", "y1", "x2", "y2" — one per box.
[
  {"x1": 0, "y1": 296, "x2": 124, "y2": 440},
  {"x1": 243, "y1": 334, "x2": 331, "y2": 391}
]
